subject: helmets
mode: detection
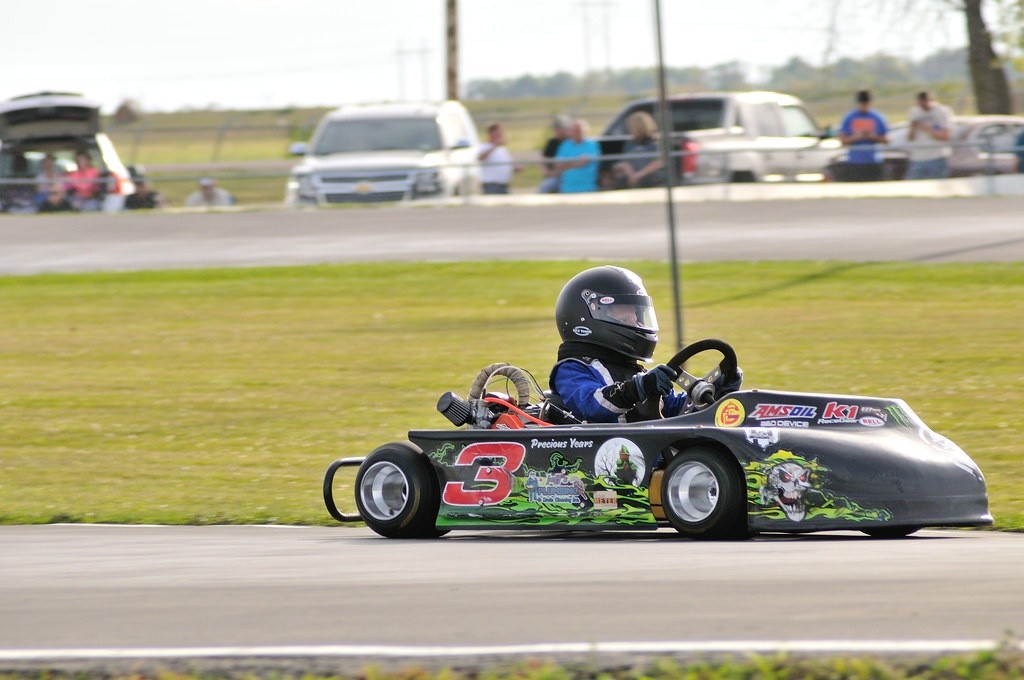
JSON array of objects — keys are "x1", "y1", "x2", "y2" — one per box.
[{"x1": 555, "y1": 265, "x2": 659, "y2": 362}]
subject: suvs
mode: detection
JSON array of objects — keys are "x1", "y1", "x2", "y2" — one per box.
[
  {"x1": 595, "y1": 93, "x2": 842, "y2": 183},
  {"x1": 0, "y1": 91, "x2": 131, "y2": 211},
  {"x1": 285, "y1": 101, "x2": 480, "y2": 204}
]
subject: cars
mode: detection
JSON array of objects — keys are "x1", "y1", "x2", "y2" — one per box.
[{"x1": 823, "y1": 118, "x2": 1023, "y2": 179}]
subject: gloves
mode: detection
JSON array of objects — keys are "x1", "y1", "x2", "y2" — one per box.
[
  {"x1": 723, "y1": 367, "x2": 743, "y2": 394},
  {"x1": 635, "y1": 364, "x2": 677, "y2": 403}
]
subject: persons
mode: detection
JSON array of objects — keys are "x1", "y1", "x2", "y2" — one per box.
[
  {"x1": 552, "y1": 118, "x2": 601, "y2": 193},
  {"x1": 549, "y1": 264, "x2": 695, "y2": 422},
  {"x1": 30, "y1": 153, "x2": 161, "y2": 214},
  {"x1": 476, "y1": 121, "x2": 523, "y2": 195},
  {"x1": 838, "y1": 89, "x2": 891, "y2": 181},
  {"x1": 901, "y1": 91, "x2": 952, "y2": 179},
  {"x1": 184, "y1": 178, "x2": 236, "y2": 209},
  {"x1": 611, "y1": 110, "x2": 666, "y2": 189},
  {"x1": 537, "y1": 114, "x2": 573, "y2": 193}
]
